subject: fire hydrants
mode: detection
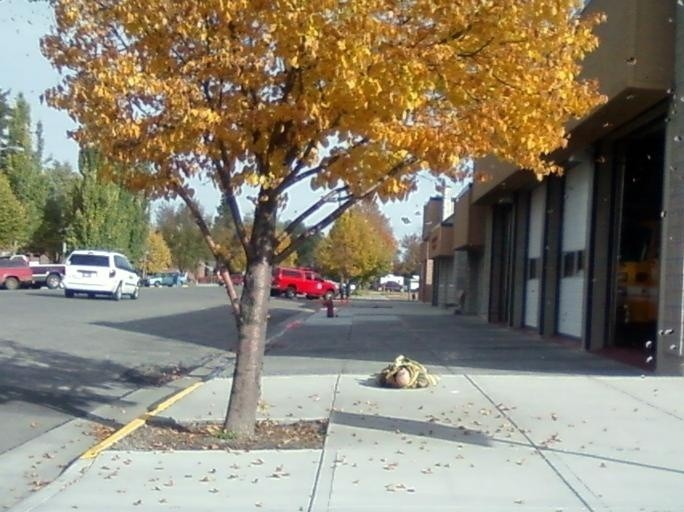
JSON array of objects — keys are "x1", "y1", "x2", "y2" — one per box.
[{"x1": 323, "y1": 297, "x2": 334, "y2": 318}]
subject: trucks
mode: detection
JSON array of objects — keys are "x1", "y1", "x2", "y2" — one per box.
[{"x1": 372, "y1": 275, "x2": 420, "y2": 292}]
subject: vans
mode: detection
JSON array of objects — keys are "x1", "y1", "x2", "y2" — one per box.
[{"x1": 271, "y1": 268, "x2": 337, "y2": 300}]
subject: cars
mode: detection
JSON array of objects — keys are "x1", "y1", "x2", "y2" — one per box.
[
  {"x1": 218, "y1": 275, "x2": 245, "y2": 289},
  {"x1": 145, "y1": 272, "x2": 187, "y2": 288},
  {"x1": 0, "y1": 249, "x2": 141, "y2": 301}
]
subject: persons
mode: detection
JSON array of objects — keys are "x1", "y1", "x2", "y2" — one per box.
[{"x1": 380, "y1": 354, "x2": 441, "y2": 389}]
